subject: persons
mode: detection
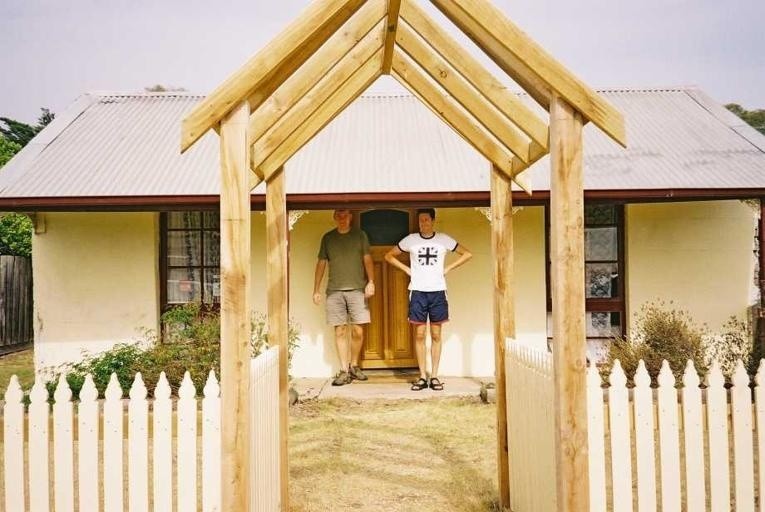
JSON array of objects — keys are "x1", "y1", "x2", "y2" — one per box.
[
  {"x1": 385, "y1": 208, "x2": 471, "y2": 390},
  {"x1": 310, "y1": 207, "x2": 375, "y2": 386}
]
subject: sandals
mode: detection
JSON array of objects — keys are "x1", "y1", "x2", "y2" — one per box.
[
  {"x1": 429, "y1": 377, "x2": 444, "y2": 390},
  {"x1": 410, "y1": 376, "x2": 428, "y2": 391}
]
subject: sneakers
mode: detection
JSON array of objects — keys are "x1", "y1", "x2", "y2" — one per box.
[
  {"x1": 350, "y1": 364, "x2": 368, "y2": 381},
  {"x1": 331, "y1": 369, "x2": 353, "y2": 385}
]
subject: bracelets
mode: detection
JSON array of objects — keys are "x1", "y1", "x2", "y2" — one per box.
[{"x1": 368, "y1": 279, "x2": 374, "y2": 283}]
